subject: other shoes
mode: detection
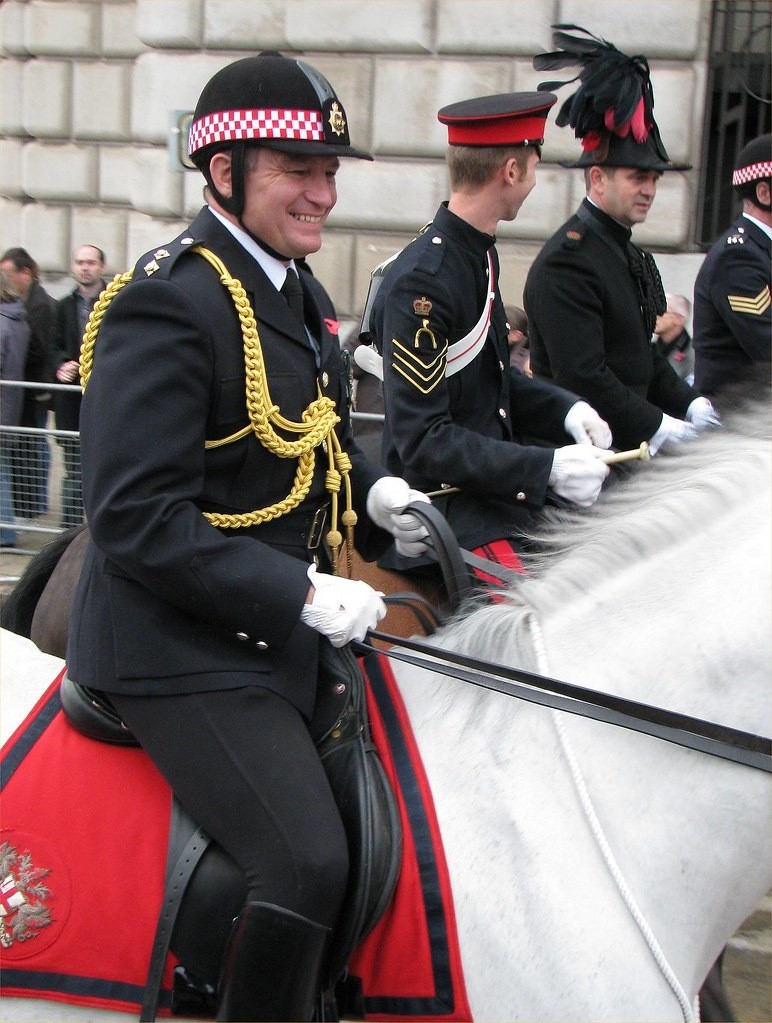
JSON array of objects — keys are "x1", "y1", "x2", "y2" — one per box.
[{"x1": 12, "y1": 515, "x2": 39, "y2": 534}]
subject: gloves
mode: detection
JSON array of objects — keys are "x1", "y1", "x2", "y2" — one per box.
[
  {"x1": 300, "y1": 561, "x2": 387, "y2": 649},
  {"x1": 547, "y1": 445, "x2": 615, "y2": 509},
  {"x1": 663, "y1": 418, "x2": 697, "y2": 452},
  {"x1": 689, "y1": 400, "x2": 720, "y2": 432},
  {"x1": 564, "y1": 399, "x2": 613, "y2": 448},
  {"x1": 366, "y1": 474, "x2": 433, "y2": 560}
]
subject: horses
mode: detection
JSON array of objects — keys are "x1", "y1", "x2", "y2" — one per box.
[{"x1": 1, "y1": 391, "x2": 772, "y2": 1023}]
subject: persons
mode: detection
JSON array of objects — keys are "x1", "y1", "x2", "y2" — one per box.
[
  {"x1": 73, "y1": 50, "x2": 436, "y2": 1023},
  {"x1": 0, "y1": 243, "x2": 107, "y2": 549},
  {"x1": 373, "y1": 90, "x2": 615, "y2": 590},
  {"x1": 504, "y1": 305, "x2": 531, "y2": 379},
  {"x1": 693, "y1": 134, "x2": 772, "y2": 392},
  {"x1": 524, "y1": 23, "x2": 721, "y2": 452},
  {"x1": 654, "y1": 294, "x2": 694, "y2": 386}
]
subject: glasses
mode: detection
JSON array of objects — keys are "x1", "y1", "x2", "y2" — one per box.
[{"x1": 665, "y1": 311, "x2": 685, "y2": 318}]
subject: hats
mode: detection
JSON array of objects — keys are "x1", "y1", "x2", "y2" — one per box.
[
  {"x1": 732, "y1": 135, "x2": 771, "y2": 211},
  {"x1": 556, "y1": 23, "x2": 693, "y2": 172},
  {"x1": 188, "y1": 48, "x2": 374, "y2": 166},
  {"x1": 437, "y1": 91, "x2": 557, "y2": 162}
]
